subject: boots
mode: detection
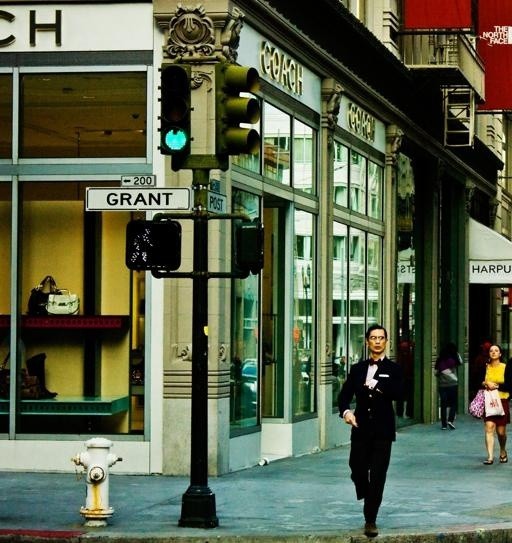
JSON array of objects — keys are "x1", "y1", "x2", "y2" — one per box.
[{"x1": 26, "y1": 350, "x2": 58, "y2": 398}]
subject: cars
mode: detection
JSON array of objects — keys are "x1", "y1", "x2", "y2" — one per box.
[{"x1": 241, "y1": 358, "x2": 355, "y2": 418}]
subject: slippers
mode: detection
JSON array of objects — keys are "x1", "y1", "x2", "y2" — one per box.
[
  {"x1": 499, "y1": 450, "x2": 508, "y2": 463},
  {"x1": 482, "y1": 460, "x2": 493, "y2": 464}
]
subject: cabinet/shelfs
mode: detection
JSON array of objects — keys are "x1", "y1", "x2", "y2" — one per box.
[{"x1": 0, "y1": 268, "x2": 145, "y2": 416}]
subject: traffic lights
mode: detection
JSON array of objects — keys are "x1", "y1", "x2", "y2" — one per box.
[
  {"x1": 126, "y1": 219, "x2": 180, "y2": 272},
  {"x1": 161, "y1": 65, "x2": 191, "y2": 155},
  {"x1": 235, "y1": 218, "x2": 264, "y2": 275},
  {"x1": 221, "y1": 65, "x2": 258, "y2": 155}
]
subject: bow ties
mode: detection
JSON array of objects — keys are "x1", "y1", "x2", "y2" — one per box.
[{"x1": 369, "y1": 357, "x2": 382, "y2": 366}]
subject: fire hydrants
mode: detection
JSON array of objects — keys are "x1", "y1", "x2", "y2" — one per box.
[{"x1": 71, "y1": 437, "x2": 122, "y2": 527}]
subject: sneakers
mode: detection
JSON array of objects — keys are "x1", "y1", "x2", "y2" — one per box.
[{"x1": 440, "y1": 421, "x2": 456, "y2": 430}]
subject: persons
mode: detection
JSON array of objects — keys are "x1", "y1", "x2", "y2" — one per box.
[
  {"x1": 471, "y1": 333, "x2": 492, "y2": 384},
  {"x1": 228, "y1": 355, "x2": 244, "y2": 420},
  {"x1": 306, "y1": 352, "x2": 345, "y2": 411},
  {"x1": 475, "y1": 344, "x2": 511, "y2": 464},
  {"x1": 338, "y1": 322, "x2": 406, "y2": 536},
  {"x1": 393, "y1": 330, "x2": 416, "y2": 419},
  {"x1": 435, "y1": 341, "x2": 463, "y2": 430}
]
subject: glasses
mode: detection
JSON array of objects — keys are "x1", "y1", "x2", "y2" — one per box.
[{"x1": 367, "y1": 335, "x2": 386, "y2": 340}]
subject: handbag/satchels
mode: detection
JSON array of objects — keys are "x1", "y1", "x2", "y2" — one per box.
[
  {"x1": 484, "y1": 389, "x2": 506, "y2": 418},
  {"x1": 46, "y1": 287, "x2": 79, "y2": 316},
  {"x1": 468, "y1": 388, "x2": 485, "y2": 418},
  {"x1": 1, "y1": 350, "x2": 27, "y2": 400},
  {"x1": 27, "y1": 274, "x2": 63, "y2": 314}
]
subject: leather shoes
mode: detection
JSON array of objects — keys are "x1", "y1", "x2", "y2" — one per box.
[{"x1": 364, "y1": 522, "x2": 379, "y2": 537}]
